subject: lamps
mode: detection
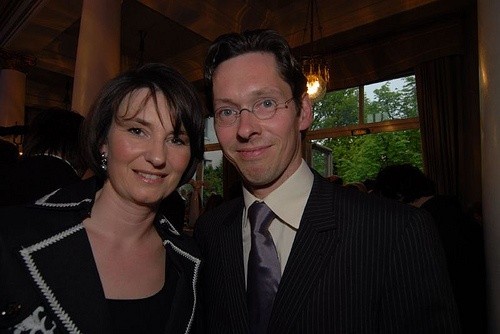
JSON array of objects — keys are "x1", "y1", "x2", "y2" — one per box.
[{"x1": 300, "y1": 0, "x2": 330, "y2": 101}]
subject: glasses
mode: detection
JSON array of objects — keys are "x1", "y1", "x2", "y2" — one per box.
[{"x1": 208, "y1": 95, "x2": 294, "y2": 126}]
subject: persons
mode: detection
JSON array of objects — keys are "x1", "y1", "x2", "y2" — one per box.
[
  {"x1": 0, "y1": 63, "x2": 207, "y2": 334},
  {"x1": 372, "y1": 162, "x2": 488, "y2": 334},
  {"x1": 191, "y1": 28, "x2": 460, "y2": 334}
]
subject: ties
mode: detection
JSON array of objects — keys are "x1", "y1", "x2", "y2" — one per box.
[{"x1": 246, "y1": 202, "x2": 281, "y2": 334}]
